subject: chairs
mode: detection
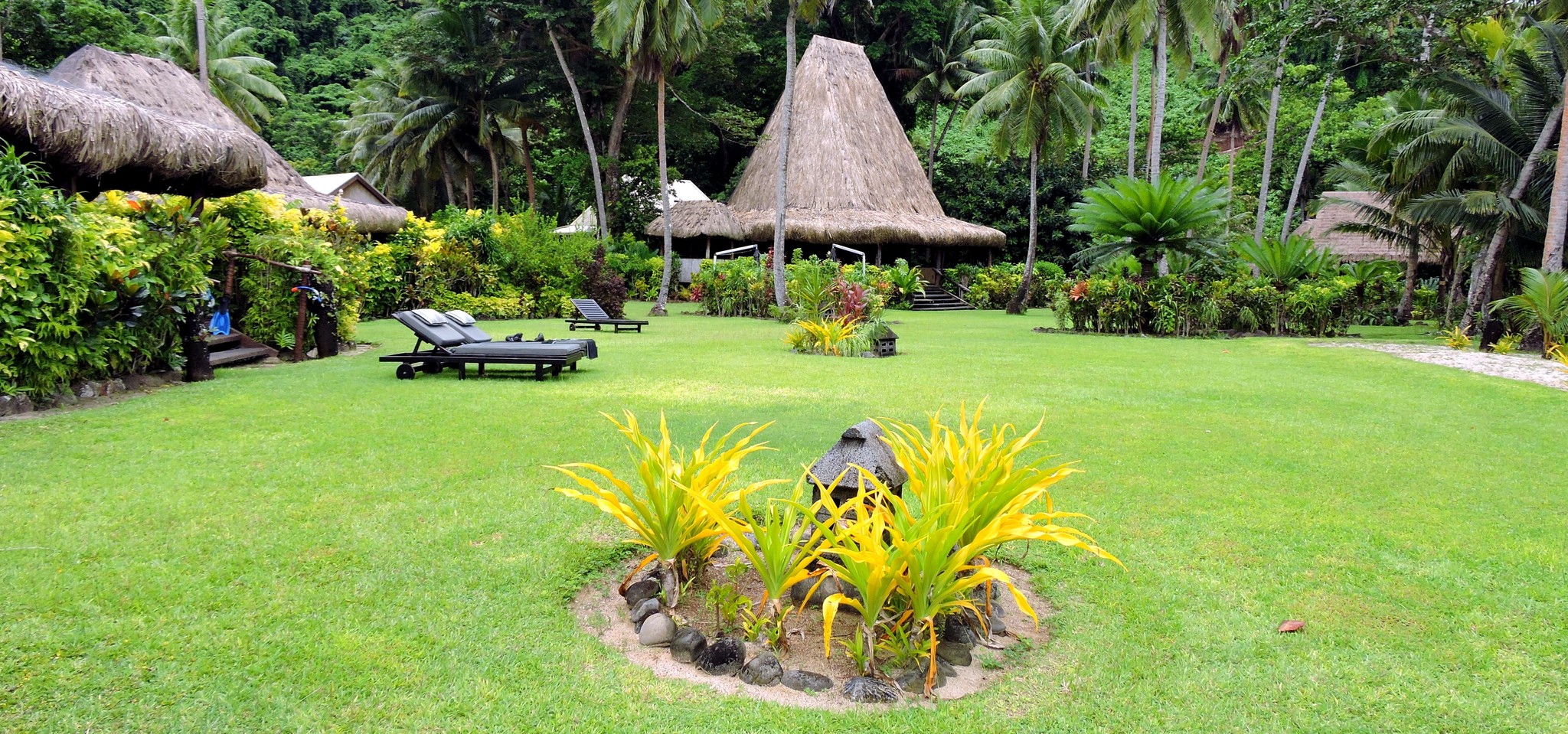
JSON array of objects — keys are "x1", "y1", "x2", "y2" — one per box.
[{"x1": 378, "y1": 299, "x2": 648, "y2": 381}]
